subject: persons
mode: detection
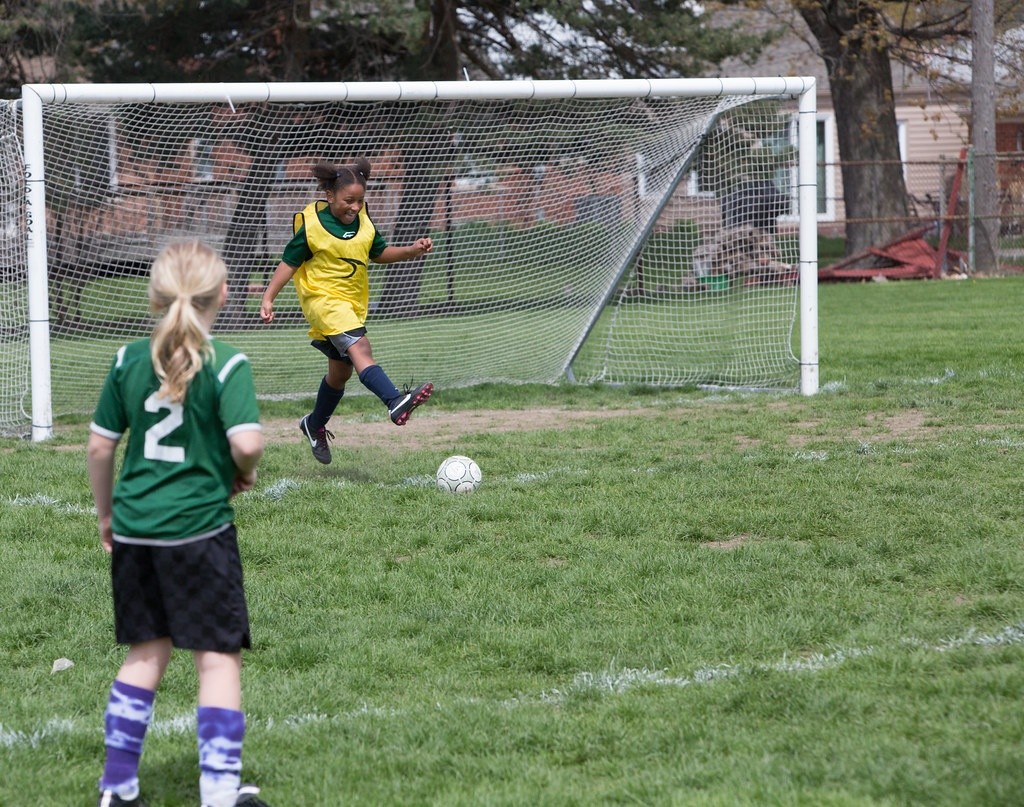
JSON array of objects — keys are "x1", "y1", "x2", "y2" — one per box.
[
  {"x1": 87, "y1": 241, "x2": 275, "y2": 807},
  {"x1": 259, "y1": 157, "x2": 435, "y2": 465}
]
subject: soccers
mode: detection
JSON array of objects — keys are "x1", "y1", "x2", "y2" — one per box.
[{"x1": 436, "y1": 455, "x2": 482, "y2": 493}]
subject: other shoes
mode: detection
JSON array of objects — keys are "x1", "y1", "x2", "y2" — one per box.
[
  {"x1": 235, "y1": 784, "x2": 269, "y2": 807},
  {"x1": 97, "y1": 788, "x2": 142, "y2": 807}
]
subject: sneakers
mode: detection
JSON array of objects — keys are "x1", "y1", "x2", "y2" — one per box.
[
  {"x1": 387, "y1": 374, "x2": 433, "y2": 426},
  {"x1": 300, "y1": 414, "x2": 336, "y2": 465}
]
